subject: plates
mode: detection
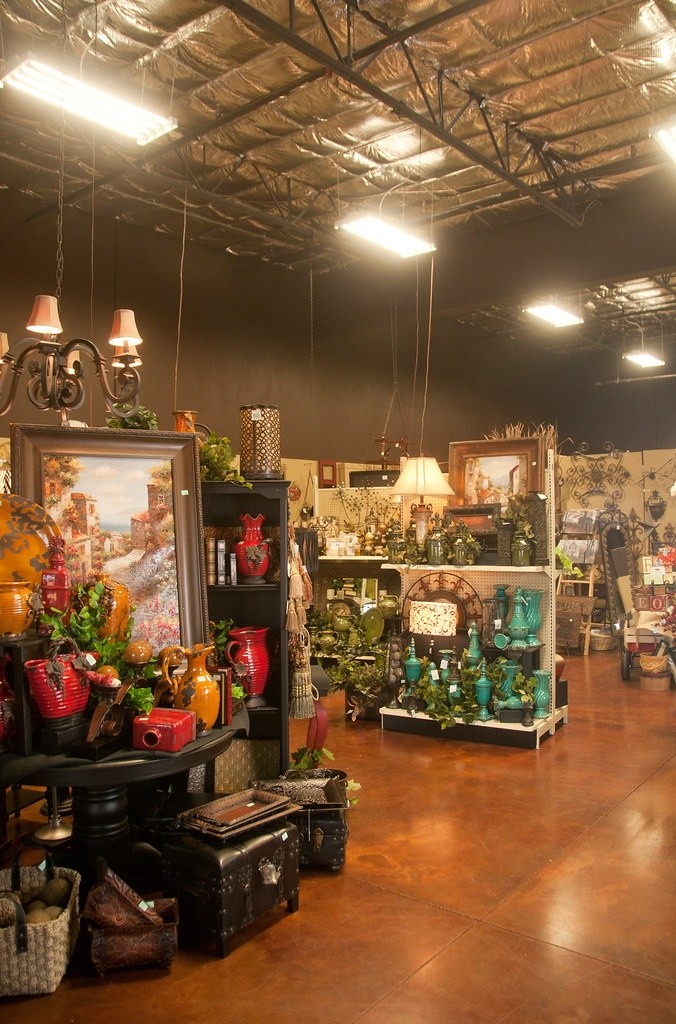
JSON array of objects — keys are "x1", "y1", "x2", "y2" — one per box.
[
  {"x1": 359, "y1": 609, "x2": 385, "y2": 644},
  {"x1": 0, "y1": 492, "x2": 63, "y2": 590}
]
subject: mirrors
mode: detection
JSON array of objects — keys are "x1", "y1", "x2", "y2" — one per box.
[{"x1": 601, "y1": 524, "x2": 634, "y2": 638}]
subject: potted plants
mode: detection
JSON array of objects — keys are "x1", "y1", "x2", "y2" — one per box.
[{"x1": 316, "y1": 646, "x2": 393, "y2": 722}]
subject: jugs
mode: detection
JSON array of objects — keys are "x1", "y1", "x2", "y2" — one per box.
[
  {"x1": 160, "y1": 643, "x2": 221, "y2": 737},
  {"x1": 225, "y1": 625, "x2": 269, "y2": 697},
  {"x1": 316, "y1": 613, "x2": 360, "y2": 653}
]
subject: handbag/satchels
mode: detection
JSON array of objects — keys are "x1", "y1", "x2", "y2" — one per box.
[
  {"x1": 640, "y1": 652, "x2": 669, "y2": 673},
  {"x1": 0, "y1": 842, "x2": 80, "y2": 995},
  {"x1": 249, "y1": 767, "x2": 349, "y2": 811}
]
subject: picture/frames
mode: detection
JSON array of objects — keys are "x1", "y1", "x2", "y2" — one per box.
[
  {"x1": 449, "y1": 438, "x2": 544, "y2": 520},
  {"x1": 10, "y1": 423, "x2": 215, "y2": 674},
  {"x1": 442, "y1": 504, "x2": 500, "y2": 535}
]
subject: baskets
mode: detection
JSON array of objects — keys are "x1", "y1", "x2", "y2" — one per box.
[{"x1": 589, "y1": 633, "x2": 617, "y2": 651}]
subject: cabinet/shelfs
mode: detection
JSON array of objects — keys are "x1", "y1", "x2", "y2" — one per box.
[
  {"x1": 380, "y1": 449, "x2": 570, "y2": 750},
  {"x1": 201, "y1": 481, "x2": 290, "y2": 796}
]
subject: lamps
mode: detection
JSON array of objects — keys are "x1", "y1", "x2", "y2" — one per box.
[
  {"x1": 410, "y1": 601, "x2": 458, "y2": 659},
  {"x1": 390, "y1": 452, "x2": 456, "y2": 544},
  {"x1": 622, "y1": 348, "x2": 665, "y2": 369},
  {"x1": 525, "y1": 299, "x2": 584, "y2": 327},
  {"x1": 0, "y1": 47, "x2": 177, "y2": 146},
  {"x1": 334, "y1": 209, "x2": 436, "y2": 258},
  {"x1": 240, "y1": 404, "x2": 282, "y2": 480},
  {"x1": 0, "y1": 0, "x2": 143, "y2": 419}
]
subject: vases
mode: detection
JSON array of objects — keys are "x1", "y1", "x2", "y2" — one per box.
[
  {"x1": 0, "y1": 582, "x2": 35, "y2": 641},
  {"x1": 226, "y1": 626, "x2": 270, "y2": 707},
  {"x1": 162, "y1": 643, "x2": 220, "y2": 735},
  {"x1": 406, "y1": 591, "x2": 552, "y2": 721},
  {"x1": 310, "y1": 614, "x2": 360, "y2": 655},
  {"x1": 236, "y1": 512, "x2": 269, "y2": 584}
]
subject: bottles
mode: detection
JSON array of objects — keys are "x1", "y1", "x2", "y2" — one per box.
[
  {"x1": 79, "y1": 571, "x2": 129, "y2": 641},
  {"x1": 0, "y1": 580, "x2": 33, "y2": 638},
  {"x1": 532, "y1": 669, "x2": 550, "y2": 718},
  {"x1": 234, "y1": 514, "x2": 270, "y2": 586},
  {"x1": 171, "y1": 409, "x2": 198, "y2": 431},
  {"x1": 499, "y1": 661, "x2": 519, "y2": 697},
  {"x1": 523, "y1": 590, "x2": 544, "y2": 644},
  {"x1": 492, "y1": 582, "x2": 510, "y2": 623}
]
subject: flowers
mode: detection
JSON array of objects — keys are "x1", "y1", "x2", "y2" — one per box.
[{"x1": 651, "y1": 593, "x2": 676, "y2": 641}]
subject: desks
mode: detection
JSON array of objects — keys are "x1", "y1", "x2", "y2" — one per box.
[{"x1": 0, "y1": 700, "x2": 252, "y2": 941}]
